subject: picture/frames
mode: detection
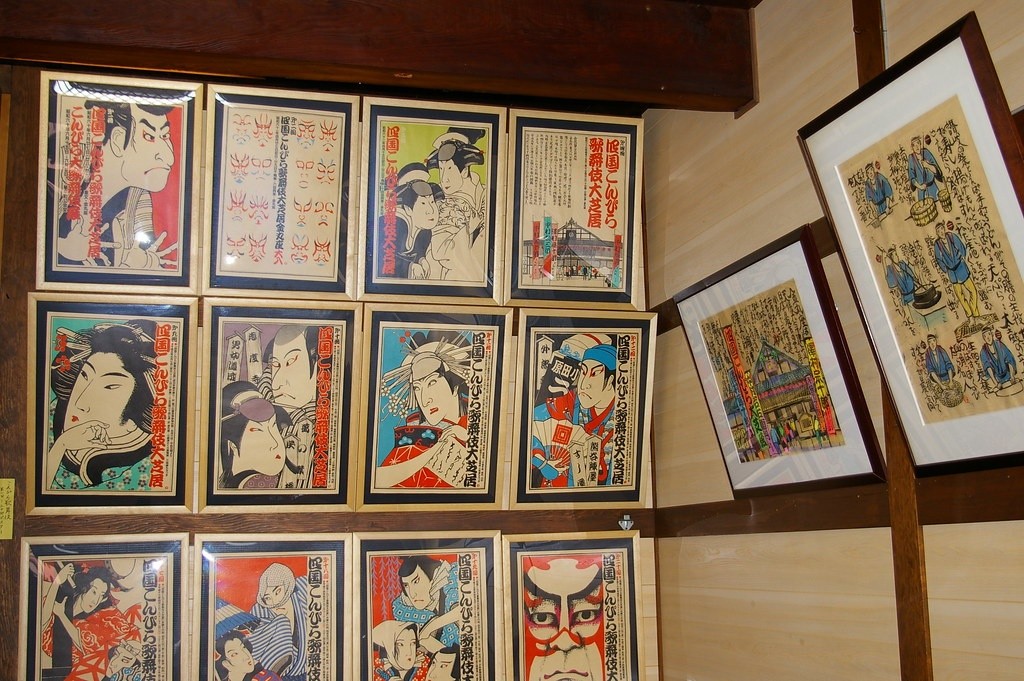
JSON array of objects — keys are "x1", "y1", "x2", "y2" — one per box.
[
  {"x1": 18, "y1": 531, "x2": 190, "y2": 681},
  {"x1": 202, "y1": 82, "x2": 359, "y2": 301},
  {"x1": 796, "y1": 11, "x2": 1024, "y2": 478},
  {"x1": 27, "y1": 292, "x2": 194, "y2": 516},
  {"x1": 511, "y1": 307, "x2": 658, "y2": 510},
  {"x1": 197, "y1": 297, "x2": 363, "y2": 513},
  {"x1": 191, "y1": 533, "x2": 351, "y2": 681},
  {"x1": 502, "y1": 530, "x2": 646, "y2": 681},
  {"x1": 351, "y1": 529, "x2": 501, "y2": 681},
  {"x1": 504, "y1": 108, "x2": 645, "y2": 311},
  {"x1": 357, "y1": 96, "x2": 507, "y2": 305},
  {"x1": 355, "y1": 303, "x2": 513, "y2": 511},
  {"x1": 35, "y1": 70, "x2": 202, "y2": 296},
  {"x1": 672, "y1": 223, "x2": 887, "y2": 502}
]
